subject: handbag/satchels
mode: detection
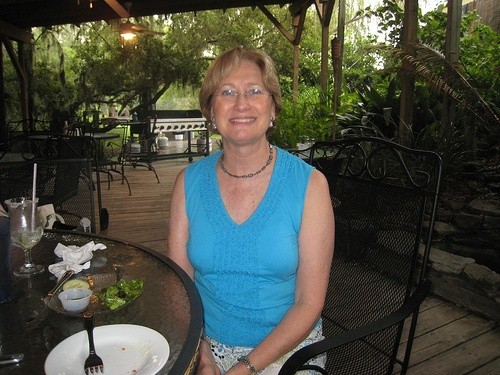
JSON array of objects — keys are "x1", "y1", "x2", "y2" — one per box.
[{"x1": 42, "y1": 213, "x2": 79, "y2": 241}]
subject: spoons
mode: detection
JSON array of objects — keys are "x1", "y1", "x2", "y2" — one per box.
[{"x1": 113, "y1": 266, "x2": 126, "y2": 298}]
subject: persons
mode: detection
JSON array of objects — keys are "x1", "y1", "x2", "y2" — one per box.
[{"x1": 167, "y1": 48, "x2": 334, "y2": 375}]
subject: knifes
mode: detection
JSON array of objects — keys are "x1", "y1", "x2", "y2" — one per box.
[{"x1": 25, "y1": 270, "x2": 75, "y2": 325}]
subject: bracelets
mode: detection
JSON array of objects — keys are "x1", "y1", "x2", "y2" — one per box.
[{"x1": 238, "y1": 356, "x2": 257, "y2": 374}]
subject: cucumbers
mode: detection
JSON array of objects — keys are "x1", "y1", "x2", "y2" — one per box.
[{"x1": 63, "y1": 280, "x2": 90, "y2": 291}]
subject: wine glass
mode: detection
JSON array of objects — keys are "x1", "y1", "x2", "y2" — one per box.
[{"x1": 4, "y1": 197, "x2": 46, "y2": 277}]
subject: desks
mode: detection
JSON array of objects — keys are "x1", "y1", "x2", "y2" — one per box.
[
  {"x1": 27, "y1": 132, "x2": 120, "y2": 181},
  {"x1": 0, "y1": 228, "x2": 204, "y2": 375},
  {"x1": 120, "y1": 121, "x2": 146, "y2": 125}
]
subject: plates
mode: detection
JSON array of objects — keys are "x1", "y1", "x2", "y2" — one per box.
[
  {"x1": 42, "y1": 273, "x2": 142, "y2": 316},
  {"x1": 44, "y1": 324, "x2": 170, "y2": 375}
]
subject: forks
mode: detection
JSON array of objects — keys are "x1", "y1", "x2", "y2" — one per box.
[{"x1": 82, "y1": 315, "x2": 103, "y2": 375}]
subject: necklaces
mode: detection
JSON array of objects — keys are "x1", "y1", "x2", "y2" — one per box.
[{"x1": 220, "y1": 143, "x2": 273, "y2": 178}]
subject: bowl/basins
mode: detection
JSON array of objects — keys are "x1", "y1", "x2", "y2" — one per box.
[{"x1": 58, "y1": 288, "x2": 92, "y2": 314}]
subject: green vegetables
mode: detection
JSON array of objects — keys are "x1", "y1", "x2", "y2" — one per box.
[{"x1": 98, "y1": 279, "x2": 145, "y2": 310}]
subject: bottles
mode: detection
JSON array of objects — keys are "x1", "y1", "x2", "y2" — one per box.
[{"x1": 63, "y1": 121, "x2": 68, "y2": 135}]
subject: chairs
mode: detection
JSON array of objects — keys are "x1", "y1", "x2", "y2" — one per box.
[
  {"x1": 0, "y1": 116, "x2": 160, "y2": 234},
  {"x1": 277, "y1": 137, "x2": 441, "y2": 375}
]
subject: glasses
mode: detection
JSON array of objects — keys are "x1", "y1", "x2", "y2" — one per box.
[{"x1": 212, "y1": 87, "x2": 272, "y2": 102}]
